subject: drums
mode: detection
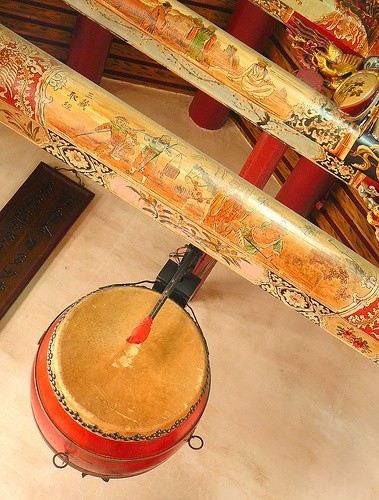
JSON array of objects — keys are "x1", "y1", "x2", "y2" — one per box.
[{"x1": 28, "y1": 282, "x2": 213, "y2": 482}]
